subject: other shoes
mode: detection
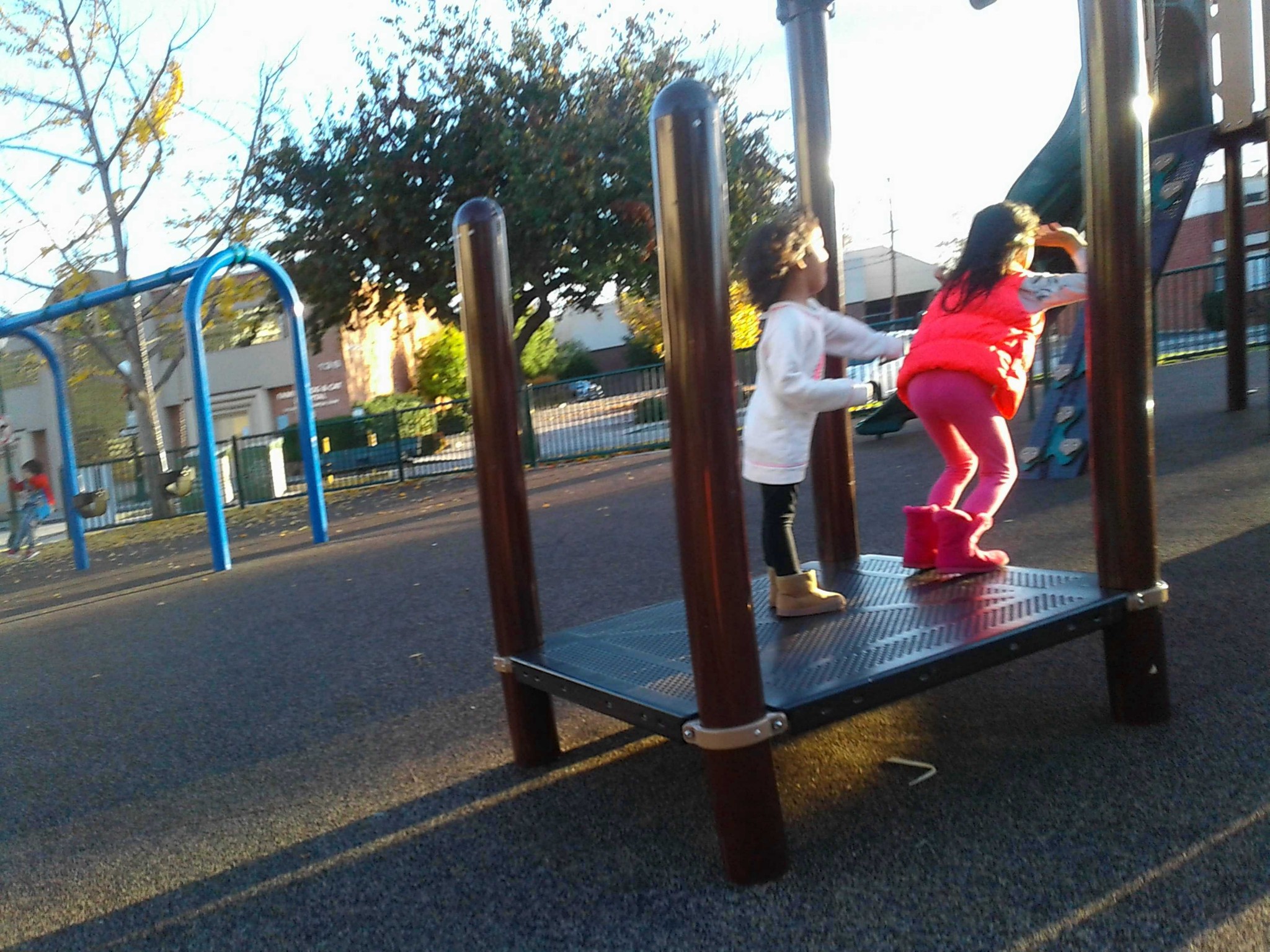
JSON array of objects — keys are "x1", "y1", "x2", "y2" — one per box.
[
  {"x1": 22, "y1": 547, "x2": 40, "y2": 561},
  {"x1": 7, "y1": 549, "x2": 22, "y2": 558}
]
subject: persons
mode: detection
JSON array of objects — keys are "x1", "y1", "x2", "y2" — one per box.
[
  {"x1": 898, "y1": 201, "x2": 1088, "y2": 573},
  {"x1": 6, "y1": 460, "x2": 55, "y2": 561},
  {"x1": 740, "y1": 210, "x2": 904, "y2": 618}
]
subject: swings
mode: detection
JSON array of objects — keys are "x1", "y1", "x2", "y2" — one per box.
[
  {"x1": 48, "y1": 301, "x2": 109, "y2": 517},
  {"x1": 133, "y1": 281, "x2": 193, "y2": 497},
  {"x1": 0, "y1": 326, "x2": 47, "y2": 521}
]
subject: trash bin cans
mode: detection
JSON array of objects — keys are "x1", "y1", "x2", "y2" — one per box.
[
  {"x1": 175, "y1": 443, "x2": 234, "y2": 513},
  {"x1": 232, "y1": 436, "x2": 285, "y2": 503}
]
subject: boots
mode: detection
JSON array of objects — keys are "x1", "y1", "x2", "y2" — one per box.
[
  {"x1": 767, "y1": 565, "x2": 776, "y2": 609},
  {"x1": 774, "y1": 570, "x2": 846, "y2": 616},
  {"x1": 902, "y1": 504, "x2": 948, "y2": 569},
  {"x1": 932, "y1": 508, "x2": 1010, "y2": 574}
]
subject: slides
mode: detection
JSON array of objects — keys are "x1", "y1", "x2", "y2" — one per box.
[{"x1": 853, "y1": 76, "x2": 1086, "y2": 437}]
